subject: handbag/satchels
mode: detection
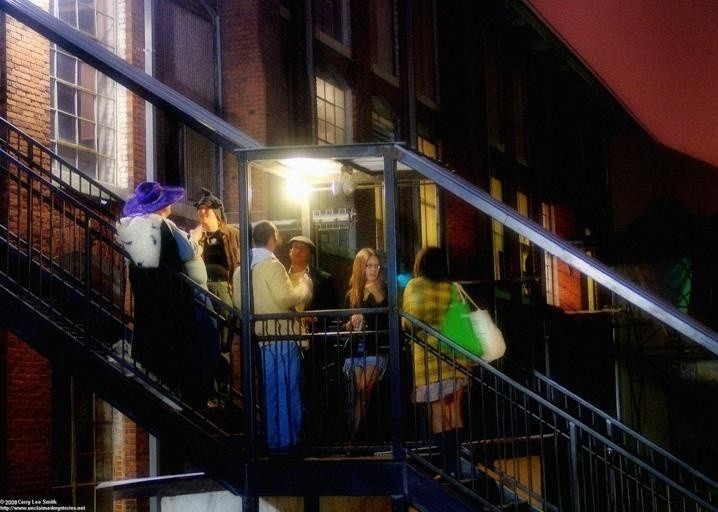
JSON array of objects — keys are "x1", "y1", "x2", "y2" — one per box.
[
  {"x1": 441, "y1": 285, "x2": 482, "y2": 359},
  {"x1": 453, "y1": 281, "x2": 506, "y2": 366}
]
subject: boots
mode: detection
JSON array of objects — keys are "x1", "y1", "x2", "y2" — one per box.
[{"x1": 433, "y1": 428, "x2": 463, "y2": 482}]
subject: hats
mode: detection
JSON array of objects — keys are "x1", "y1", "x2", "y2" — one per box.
[
  {"x1": 288, "y1": 235, "x2": 316, "y2": 254},
  {"x1": 198, "y1": 188, "x2": 222, "y2": 210},
  {"x1": 123, "y1": 182, "x2": 185, "y2": 217}
]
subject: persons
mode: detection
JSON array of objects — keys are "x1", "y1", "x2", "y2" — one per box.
[
  {"x1": 231, "y1": 222, "x2": 313, "y2": 452},
  {"x1": 400, "y1": 245, "x2": 473, "y2": 483},
  {"x1": 120, "y1": 181, "x2": 222, "y2": 415},
  {"x1": 284, "y1": 234, "x2": 336, "y2": 457},
  {"x1": 338, "y1": 248, "x2": 388, "y2": 457},
  {"x1": 187, "y1": 186, "x2": 241, "y2": 411}
]
